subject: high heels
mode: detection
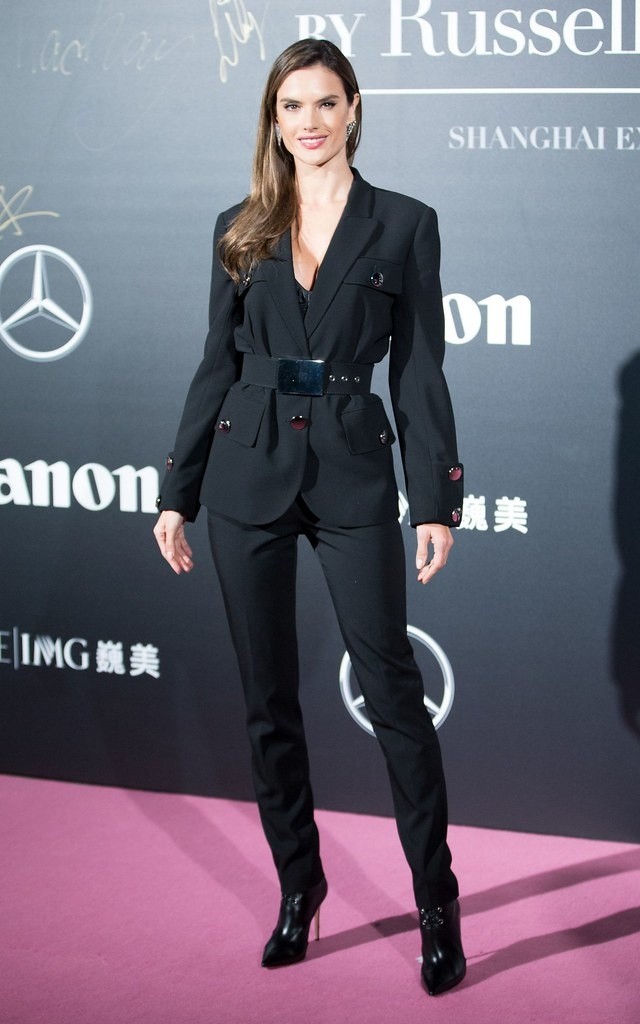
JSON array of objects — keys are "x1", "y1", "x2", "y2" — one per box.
[
  {"x1": 259, "y1": 878, "x2": 328, "y2": 965},
  {"x1": 418, "y1": 898, "x2": 466, "y2": 995}
]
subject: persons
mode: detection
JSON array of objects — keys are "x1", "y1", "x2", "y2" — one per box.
[{"x1": 149, "y1": 37, "x2": 472, "y2": 997}]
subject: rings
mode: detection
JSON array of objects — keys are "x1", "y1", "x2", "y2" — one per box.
[{"x1": 426, "y1": 561, "x2": 432, "y2": 569}]
